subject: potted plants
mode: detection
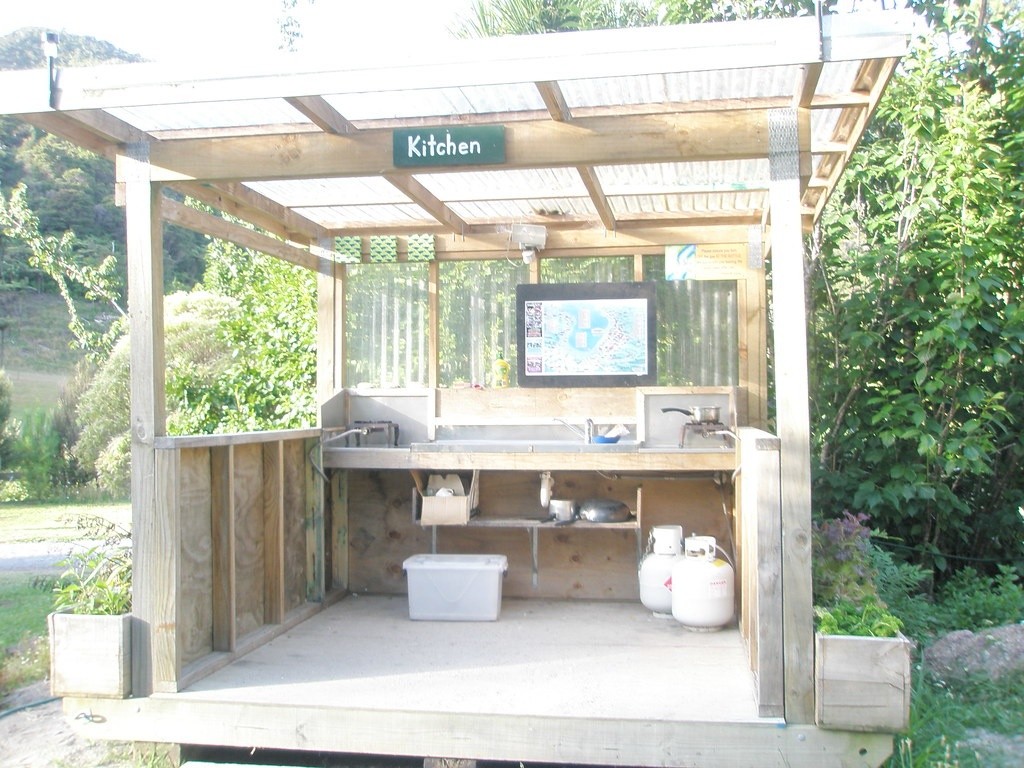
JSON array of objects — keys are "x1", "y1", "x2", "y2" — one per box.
[
  {"x1": 811, "y1": 600, "x2": 913, "y2": 735},
  {"x1": 16, "y1": 512, "x2": 134, "y2": 700}
]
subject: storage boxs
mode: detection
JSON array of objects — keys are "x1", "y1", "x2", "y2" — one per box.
[
  {"x1": 408, "y1": 469, "x2": 480, "y2": 527},
  {"x1": 403, "y1": 552, "x2": 509, "y2": 623}
]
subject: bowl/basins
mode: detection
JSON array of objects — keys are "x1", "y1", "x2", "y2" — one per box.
[{"x1": 593, "y1": 436, "x2": 620, "y2": 443}]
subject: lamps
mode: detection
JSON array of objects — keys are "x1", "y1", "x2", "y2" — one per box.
[{"x1": 510, "y1": 224, "x2": 547, "y2": 253}]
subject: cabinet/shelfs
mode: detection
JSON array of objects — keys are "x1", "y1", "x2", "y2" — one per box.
[{"x1": 408, "y1": 482, "x2": 645, "y2": 589}]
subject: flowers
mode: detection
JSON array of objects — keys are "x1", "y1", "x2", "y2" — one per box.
[{"x1": 814, "y1": 508, "x2": 875, "y2": 605}]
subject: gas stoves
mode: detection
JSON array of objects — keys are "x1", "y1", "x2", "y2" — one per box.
[{"x1": 680, "y1": 421, "x2": 739, "y2": 447}]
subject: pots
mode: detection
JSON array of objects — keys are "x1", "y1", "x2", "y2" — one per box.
[
  {"x1": 661, "y1": 406, "x2": 721, "y2": 425},
  {"x1": 555, "y1": 498, "x2": 631, "y2": 526},
  {"x1": 541, "y1": 500, "x2": 572, "y2": 523}
]
